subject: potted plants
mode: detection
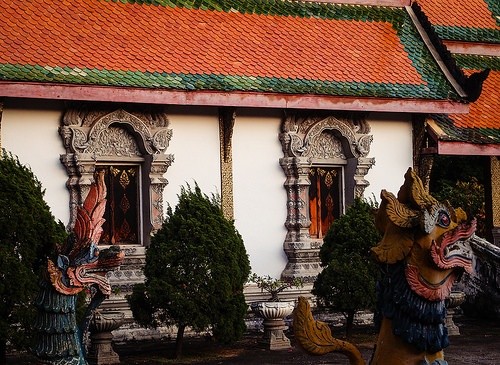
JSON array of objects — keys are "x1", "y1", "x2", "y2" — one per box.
[{"x1": 247, "y1": 273, "x2": 304, "y2": 320}]
